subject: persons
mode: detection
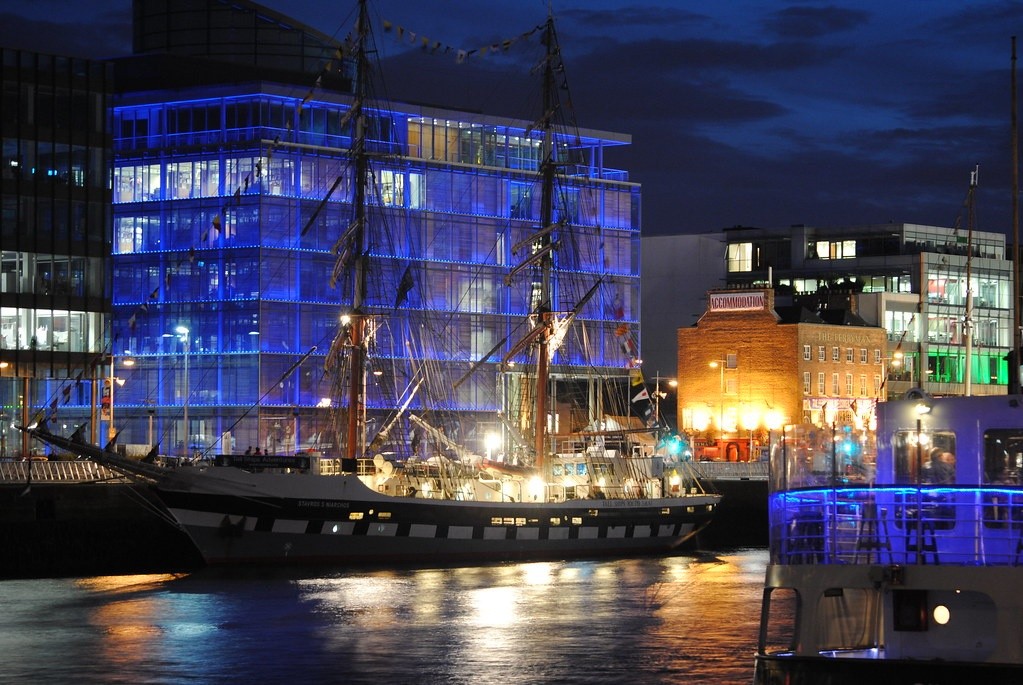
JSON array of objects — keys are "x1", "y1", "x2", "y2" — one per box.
[
  {"x1": 264, "y1": 449, "x2": 268, "y2": 456},
  {"x1": 245, "y1": 446, "x2": 252, "y2": 455},
  {"x1": 921, "y1": 448, "x2": 952, "y2": 483},
  {"x1": 254, "y1": 446, "x2": 261, "y2": 455}
]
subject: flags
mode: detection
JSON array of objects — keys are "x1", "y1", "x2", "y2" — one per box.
[
  {"x1": 35, "y1": 15, "x2": 361, "y2": 424},
  {"x1": 383, "y1": 18, "x2": 544, "y2": 64},
  {"x1": 552, "y1": 44, "x2": 674, "y2": 452}
]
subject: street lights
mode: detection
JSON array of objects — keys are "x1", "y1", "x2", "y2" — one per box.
[
  {"x1": 651, "y1": 368, "x2": 678, "y2": 443},
  {"x1": 174, "y1": 326, "x2": 190, "y2": 458},
  {"x1": 709, "y1": 354, "x2": 728, "y2": 438}
]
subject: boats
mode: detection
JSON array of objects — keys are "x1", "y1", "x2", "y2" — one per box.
[{"x1": 73, "y1": 569, "x2": 192, "y2": 602}]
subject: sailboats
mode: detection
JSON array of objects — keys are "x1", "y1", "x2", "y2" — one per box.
[
  {"x1": 751, "y1": 164, "x2": 1022, "y2": 685},
  {"x1": 1, "y1": 0, "x2": 725, "y2": 566}
]
